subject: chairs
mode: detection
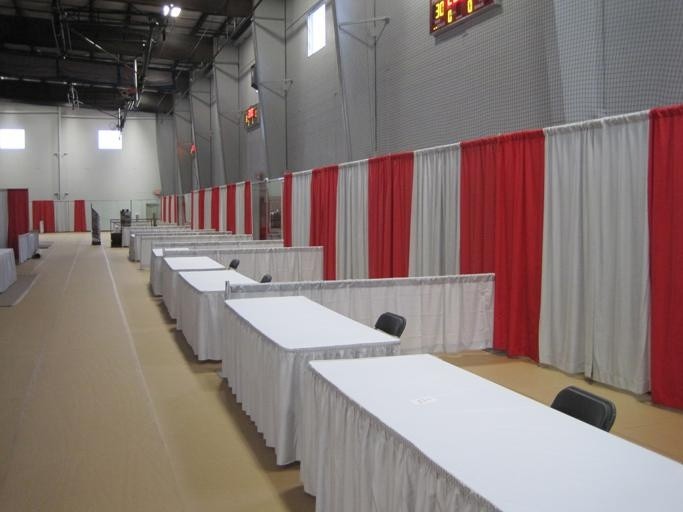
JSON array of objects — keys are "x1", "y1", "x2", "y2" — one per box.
[
  {"x1": 260, "y1": 274, "x2": 272, "y2": 283},
  {"x1": 229, "y1": 259, "x2": 240, "y2": 269},
  {"x1": 375, "y1": 312, "x2": 407, "y2": 338},
  {"x1": 550, "y1": 384, "x2": 617, "y2": 433}
]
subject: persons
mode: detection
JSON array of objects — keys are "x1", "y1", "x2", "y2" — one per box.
[
  {"x1": 120, "y1": 208, "x2": 124, "y2": 230},
  {"x1": 123, "y1": 209, "x2": 131, "y2": 227}
]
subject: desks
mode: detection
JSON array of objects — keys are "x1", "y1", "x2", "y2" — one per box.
[
  {"x1": 298, "y1": 353, "x2": 683, "y2": 511},
  {"x1": 149, "y1": 248, "x2": 192, "y2": 296},
  {"x1": 161, "y1": 257, "x2": 225, "y2": 319},
  {"x1": 178, "y1": 270, "x2": 261, "y2": 361},
  {"x1": 218, "y1": 295, "x2": 402, "y2": 467},
  {"x1": 0, "y1": 248, "x2": 17, "y2": 293}
]
